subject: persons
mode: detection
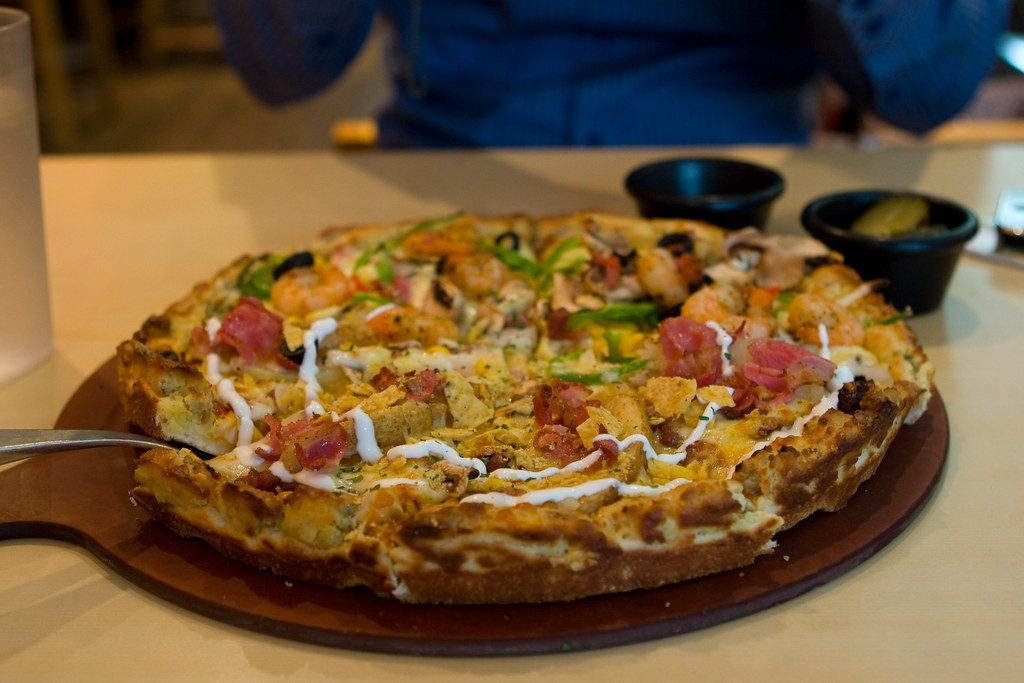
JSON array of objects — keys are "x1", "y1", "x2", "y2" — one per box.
[{"x1": 217, "y1": 0, "x2": 1012, "y2": 149}]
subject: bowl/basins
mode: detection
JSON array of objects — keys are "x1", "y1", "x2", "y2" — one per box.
[
  {"x1": 624, "y1": 156, "x2": 786, "y2": 233},
  {"x1": 800, "y1": 188, "x2": 980, "y2": 316}
]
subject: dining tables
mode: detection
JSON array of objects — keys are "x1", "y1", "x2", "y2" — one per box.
[{"x1": 0, "y1": 136, "x2": 1024, "y2": 683}]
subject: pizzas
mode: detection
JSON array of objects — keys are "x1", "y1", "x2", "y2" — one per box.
[{"x1": 120, "y1": 208, "x2": 937, "y2": 606}]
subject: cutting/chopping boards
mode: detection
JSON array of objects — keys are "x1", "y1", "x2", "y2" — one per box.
[{"x1": 0, "y1": 354, "x2": 951, "y2": 658}]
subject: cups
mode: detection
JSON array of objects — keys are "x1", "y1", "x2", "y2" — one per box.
[{"x1": 0, "y1": 8, "x2": 54, "y2": 385}]
